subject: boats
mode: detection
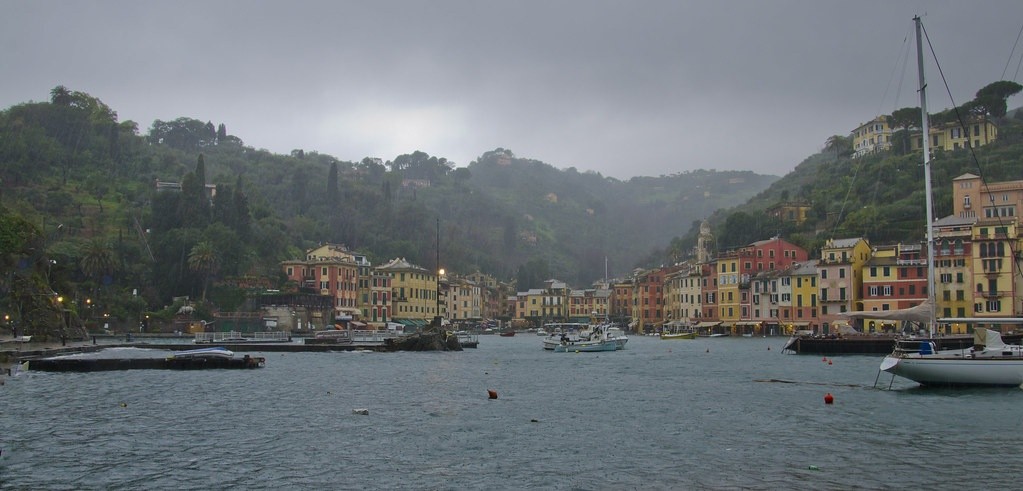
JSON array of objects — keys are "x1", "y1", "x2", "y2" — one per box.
[
  {"x1": 661, "y1": 331, "x2": 696, "y2": 339},
  {"x1": 555, "y1": 338, "x2": 616, "y2": 352},
  {"x1": 498, "y1": 330, "x2": 516, "y2": 338},
  {"x1": 537, "y1": 328, "x2": 547, "y2": 337},
  {"x1": 543, "y1": 321, "x2": 630, "y2": 351},
  {"x1": 781, "y1": 323, "x2": 1023, "y2": 354},
  {"x1": 171, "y1": 347, "x2": 235, "y2": 357}
]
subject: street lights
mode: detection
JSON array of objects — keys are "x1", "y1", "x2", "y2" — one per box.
[{"x1": 435, "y1": 269, "x2": 445, "y2": 315}]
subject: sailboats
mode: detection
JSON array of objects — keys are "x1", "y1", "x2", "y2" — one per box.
[{"x1": 878, "y1": 14, "x2": 1023, "y2": 388}]
